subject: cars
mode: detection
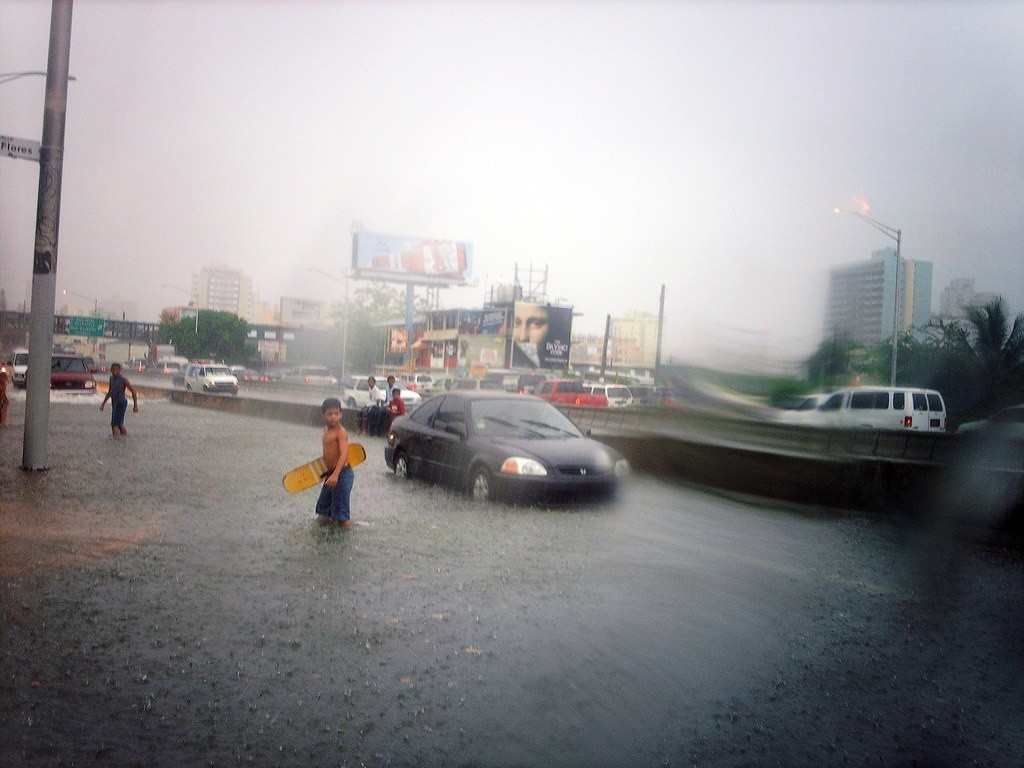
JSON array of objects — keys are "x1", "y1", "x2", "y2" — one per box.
[
  {"x1": 233, "y1": 369, "x2": 269, "y2": 382},
  {"x1": 342, "y1": 376, "x2": 422, "y2": 410},
  {"x1": 173, "y1": 368, "x2": 186, "y2": 387},
  {"x1": 385, "y1": 392, "x2": 630, "y2": 507},
  {"x1": 84, "y1": 358, "x2": 181, "y2": 375},
  {"x1": 955, "y1": 405, "x2": 1024, "y2": 440},
  {"x1": 23, "y1": 353, "x2": 97, "y2": 397},
  {"x1": 424, "y1": 377, "x2": 510, "y2": 394},
  {"x1": 280, "y1": 365, "x2": 333, "y2": 386}
]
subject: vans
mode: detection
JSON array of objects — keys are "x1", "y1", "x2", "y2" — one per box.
[
  {"x1": 399, "y1": 373, "x2": 434, "y2": 394},
  {"x1": 627, "y1": 385, "x2": 672, "y2": 407},
  {"x1": 772, "y1": 386, "x2": 949, "y2": 434},
  {"x1": 185, "y1": 365, "x2": 239, "y2": 397},
  {"x1": 581, "y1": 382, "x2": 633, "y2": 407},
  {"x1": 6, "y1": 350, "x2": 30, "y2": 390}
]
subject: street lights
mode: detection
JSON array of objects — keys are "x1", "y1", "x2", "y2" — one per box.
[
  {"x1": 834, "y1": 206, "x2": 902, "y2": 385},
  {"x1": 63, "y1": 289, "x2": 97, "y2": 353}
]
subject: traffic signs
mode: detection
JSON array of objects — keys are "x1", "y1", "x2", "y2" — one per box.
[{"x1": 70, "y1": 316, "x2": 104, "y2": 337}]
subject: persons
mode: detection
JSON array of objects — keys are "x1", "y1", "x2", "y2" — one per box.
[
  {"x1": 357, "y1": 376, "x2": 382, "y2": 436},
  {"x1": 314, "y1": 398, "x2": 355, "y2": 526},
  {"x1": 514, "y1": 304, "x2": 551, "y2": 356},
  {"x1": 387, "y1": 388, "x2": 405, "y2": 421},
  {"x1": 99, "y1": 363, "x2": 138, "y2": 436},
  {"x1": 370, "y1": 376, "x2": 398, "y2": 437}
]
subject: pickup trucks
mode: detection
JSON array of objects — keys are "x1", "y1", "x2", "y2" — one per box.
[{"x1": 536, "y1": 377, "x2": 609, "y2": 407}]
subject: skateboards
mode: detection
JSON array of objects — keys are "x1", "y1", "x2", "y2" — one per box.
[{"x1": 282, "y1": 443, "x2": 367, "y2": 495}]
